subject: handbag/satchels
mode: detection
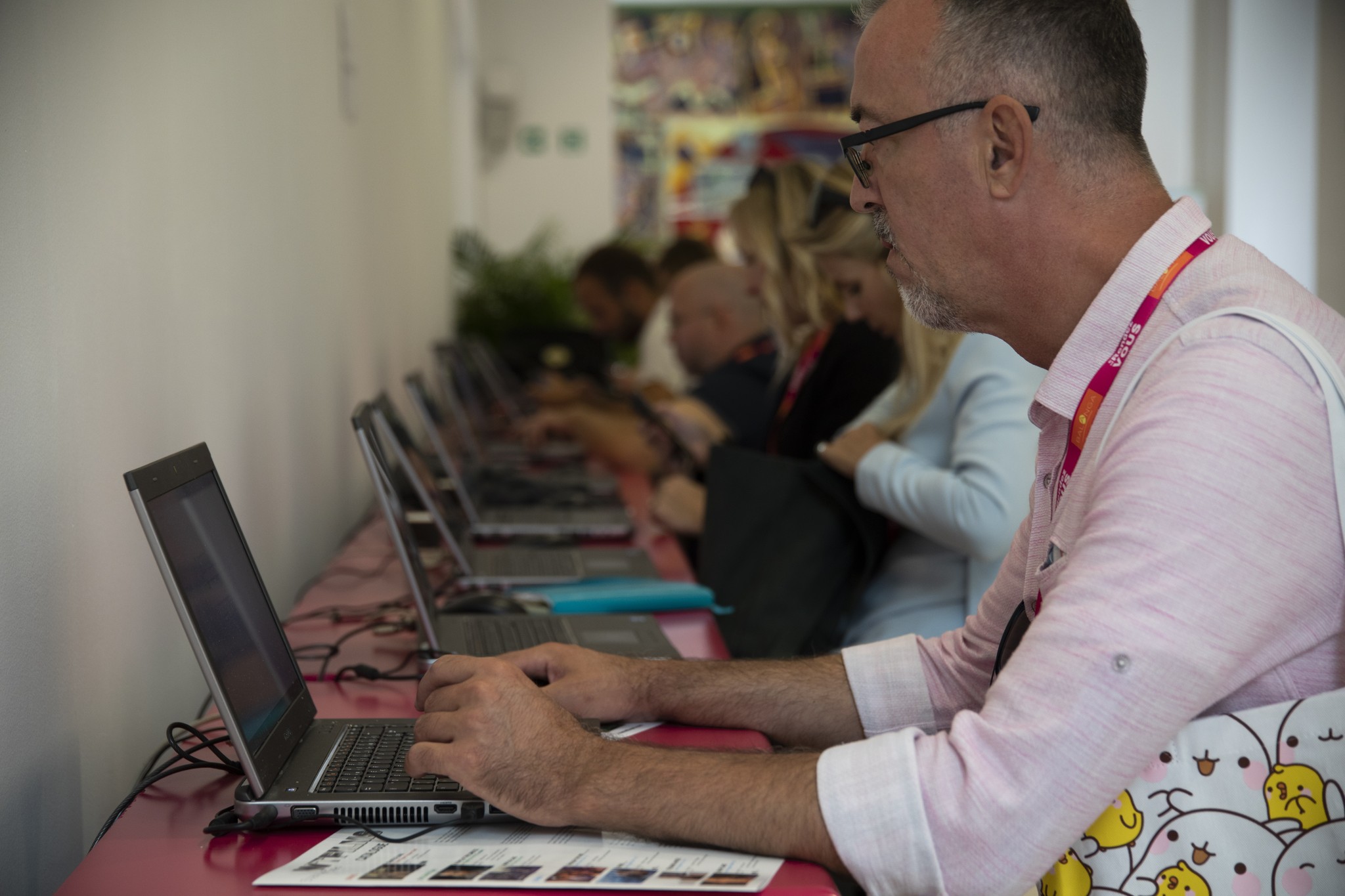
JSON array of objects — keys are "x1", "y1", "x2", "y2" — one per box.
[{"x1": 1021, "y1": 306, "x2": 1345, "y2": 896}]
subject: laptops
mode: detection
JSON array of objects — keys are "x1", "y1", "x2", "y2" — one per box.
[
  {"x1": 369, "y1": 392, "x2": 664, "y2": 589},
  {"x1": 121, "y1": 443, "x2": 601, "y2": 834},
  {"x1": 431, "y1": 345, "x2": 624, "y2": 509},
  {"x1": 462, "y1": 333, "x2": 535, "y2": 422},
  {"x1": 404, "y1": 370, "x2": 633, "y2": 545},
  {"x1": 349, "y1": 398, "x2": 683, "y2": 688}
]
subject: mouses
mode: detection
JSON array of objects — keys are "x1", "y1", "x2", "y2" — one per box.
[{"x1": 438, "y1": 590, "x2": 528, "y2": 617}]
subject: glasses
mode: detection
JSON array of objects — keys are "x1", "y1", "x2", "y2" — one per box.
[{"x1": 837, "y1": 100, "x2": 1039, "y2": 190}]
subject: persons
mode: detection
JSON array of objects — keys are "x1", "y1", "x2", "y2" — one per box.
[
  {"x1": 515, "y1": 157, "x2": 914, "y2": 647},
  {"x1": 403, "y1": 0, "x2": 1345, "y2": 896},
  {"x1": 799, "y1": 154, "x2": 1049, "y2": 656}
]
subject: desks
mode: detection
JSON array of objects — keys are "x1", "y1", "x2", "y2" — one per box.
[{"x1": 42, "y1": 397, "x2": 841, "y2": 891}]
